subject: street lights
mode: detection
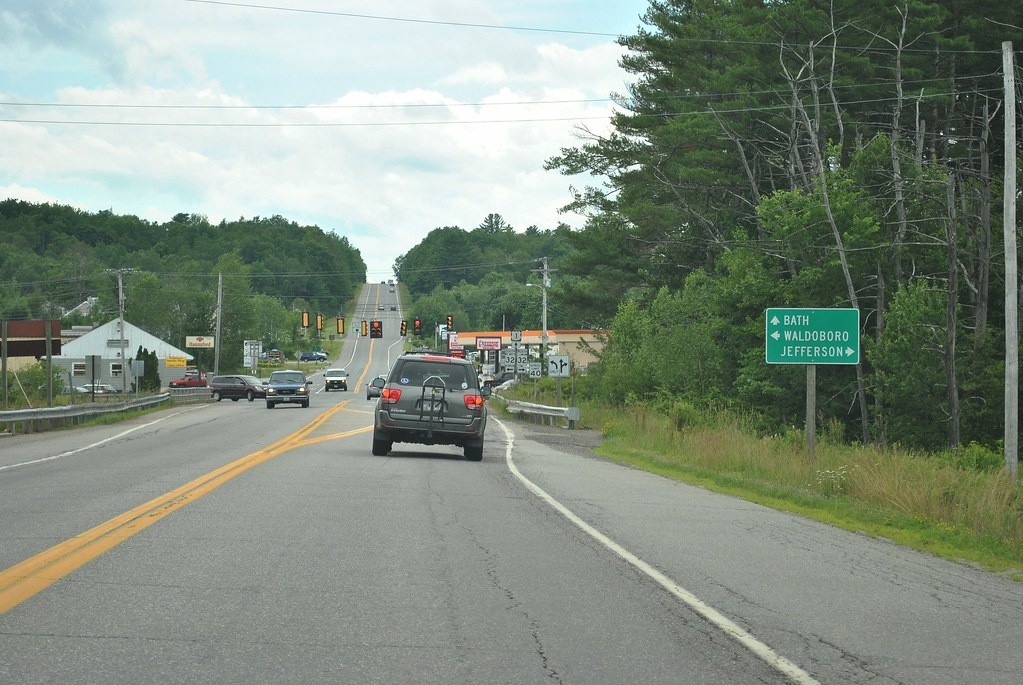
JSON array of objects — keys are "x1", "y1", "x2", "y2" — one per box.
[{"x1": 525, "y1": 283, "x2": 547, "y2": 377}]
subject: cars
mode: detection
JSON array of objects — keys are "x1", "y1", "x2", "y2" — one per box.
[
  {"x1": 378, "y1": 305, "x2": 384, "y2": 310},
  {"x1": 381, "y1": 280, "x2": 394, "y2": 293},
  {"x1": 65, "y1": 383, "x2": 118, "y2": 394},
  {"x1": 262, "y1": 369, "x2": 313, "y2": 409},
  {"x1": 391, "y1": 306, "x2": 396, "y2": 311},
  {"x1": 366, "y1": 377, "x2": 386, "y2": 400}
]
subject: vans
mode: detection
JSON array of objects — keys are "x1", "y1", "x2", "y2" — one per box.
[{"x1": 210, "y1": 375, "x2": 267, "y2": 402}]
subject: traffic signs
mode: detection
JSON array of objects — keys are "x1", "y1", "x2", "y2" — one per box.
[
  {"x1": 765, "y1": 308, "x2": 860, "y2": 365},
  {"x1": 505, "y1": 348, "x2": 527, "y2": 373}
]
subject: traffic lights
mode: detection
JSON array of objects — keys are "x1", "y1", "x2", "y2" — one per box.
[
  {"x1": 415, "y1": 321, "x2": 421, "y2": 335},
  {"x1": 401, "y1": 320, "x2": 407, "y2": 336},
  {"x1": 447, "y1": 315, "x2": 454, "y2": 331},
  {"x1": 370, "y1": 321, "x2": 382, "y2": 338}
]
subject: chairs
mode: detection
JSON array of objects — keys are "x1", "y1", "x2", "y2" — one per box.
[
  {"x1": 445, "y1": 367, "x2": 465, "y2": 386},
  {"x1": 402, "y1": 364, "x2": 424, "y2": 385}
]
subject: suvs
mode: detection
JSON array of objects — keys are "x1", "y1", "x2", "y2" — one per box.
[
  {"x1": 323, "y1": 368, "x2": 349, "y2": 391},
  {"x1": 169, "y1": 374, "x2": 207, "y2": 388},
  {"x1": 484, "y1": 372, "x2": 525, "y2": 388},
  {"x1": 301, "y1": 352, "x2": 329, "y2": 363},
  {"x1": 372, "y1": 352, "x2": 492, "y2": 461}
]
